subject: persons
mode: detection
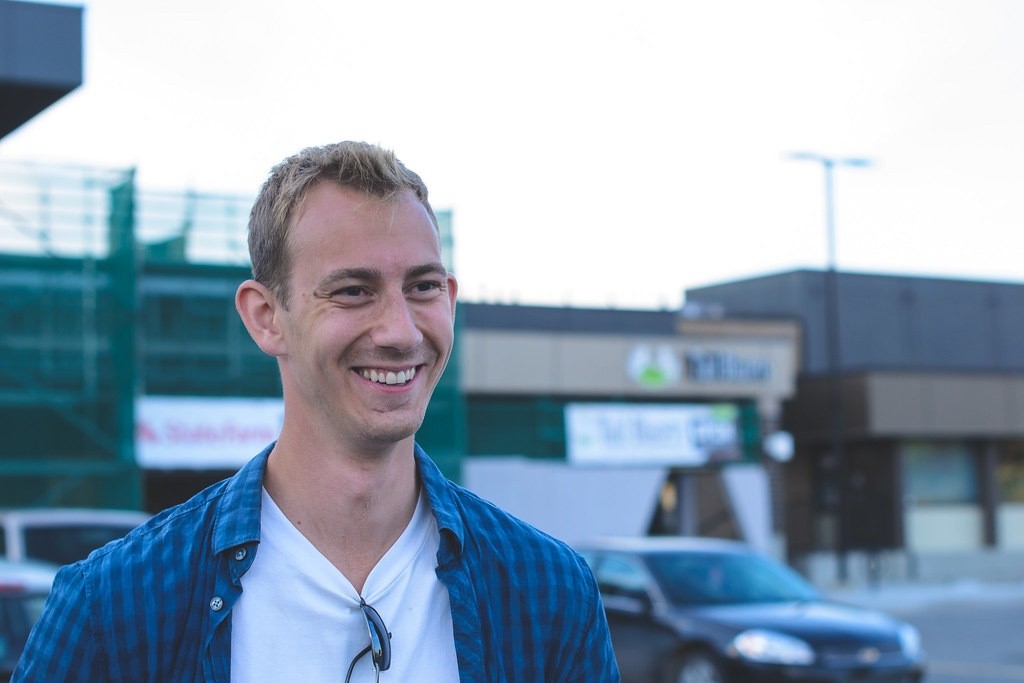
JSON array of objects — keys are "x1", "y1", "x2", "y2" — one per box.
[{"x1": 10, "y1": 140, "x2": 621, "y2": 683}]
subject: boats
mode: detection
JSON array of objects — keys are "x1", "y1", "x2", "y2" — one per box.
[{"x1": 570, "y1": 536, "x2": 926, "y2": 683}]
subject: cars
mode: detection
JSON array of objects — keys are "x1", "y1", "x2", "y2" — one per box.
[
  {"x1": 0, "y1": 509, "x2": 150, "y2": 571},
  {"x1": 0, "y1": 560, "x2": 61, "y2": 682}
]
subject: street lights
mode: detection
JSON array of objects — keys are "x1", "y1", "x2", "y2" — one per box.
[{"x1": 790, "y1": 152, "x2": 868, "y2": 585}]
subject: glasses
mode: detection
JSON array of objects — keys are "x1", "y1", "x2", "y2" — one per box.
[{"x1": 343, "y1": 596, "x2": 392, "y2": 683}]
